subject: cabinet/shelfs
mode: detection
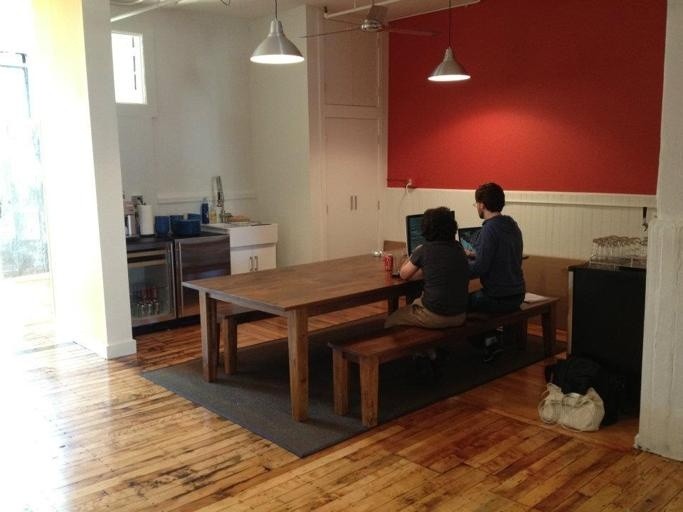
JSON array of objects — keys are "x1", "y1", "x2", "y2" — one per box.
[{"x1": 201, "y1": 221, "x2": 278, "y2": 274}]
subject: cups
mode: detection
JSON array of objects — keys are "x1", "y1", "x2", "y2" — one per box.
[
  {"x1": 589, "y1": 233, "x2": 646, "y2": 266},
  {"x1": 136, "y1": 205, "x2": 154, "y2": 236}
]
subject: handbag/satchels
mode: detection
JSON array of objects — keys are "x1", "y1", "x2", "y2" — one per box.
[
  {"x1": 536, "y1": 384, "x2": 605, "y2": 433},
  {"x1": 544, "y1": 357, "x2": 621, "y2": 426}
]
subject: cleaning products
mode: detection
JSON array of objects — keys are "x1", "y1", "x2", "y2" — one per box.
[{"x1": 201, "y1": 196, "x2": 209, "y2": 224}]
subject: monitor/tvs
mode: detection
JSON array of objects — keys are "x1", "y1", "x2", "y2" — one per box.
[
  {"x1": 405, "y1": 211, "x2": 454, "y2": 259},
  {"x1": 457, "y1": 227, "x2": 481, "y2": 258}
]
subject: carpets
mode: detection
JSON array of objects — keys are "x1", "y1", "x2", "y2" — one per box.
[{"x1": 140, "y1": 307, "x2": 566, "y2": 459}]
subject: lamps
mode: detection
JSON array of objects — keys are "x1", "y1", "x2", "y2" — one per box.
[
  {"x1": 428, "y1": 0, "x2": 472, "y2": 82},
  {"x1": 248, "y1": 0, "x2": 306, "y2": 64}
]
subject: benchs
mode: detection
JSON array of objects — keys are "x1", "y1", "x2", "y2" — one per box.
[
  {"x1": 213, "y1": 307, "x2": 279, "y2": 376},
  {"x1": 326, "y1": 291, "x2": 559, "y2": 429}
]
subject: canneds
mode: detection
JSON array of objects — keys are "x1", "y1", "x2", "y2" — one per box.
[{"x1": 383, "y1": 254, "x2": 394, "y2": 271}]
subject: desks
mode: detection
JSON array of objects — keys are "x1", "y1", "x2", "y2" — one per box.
[{"x1": 179, "y1": 245, "x2": 529, "y2": 424}]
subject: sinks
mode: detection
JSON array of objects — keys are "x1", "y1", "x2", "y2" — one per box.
[{"x1": 201, "y1": 221, "x2": 278, "y2": 248}]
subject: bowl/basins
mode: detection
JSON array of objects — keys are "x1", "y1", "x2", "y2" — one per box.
[
  {"x1": 174, "y1": 218, "x2": 199, "y2": 237},
  {"x1": 154, "y1": 213, "x2": 200, "y2": 235}
]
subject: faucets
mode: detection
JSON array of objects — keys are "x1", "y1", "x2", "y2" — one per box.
[{"x1": 216, "y1": 174, "x2": 225, "y2": 223}]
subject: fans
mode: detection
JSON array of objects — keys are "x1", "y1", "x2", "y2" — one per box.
[{"x1": 300, "y1": 1, "x2": 438, "y2": 40}]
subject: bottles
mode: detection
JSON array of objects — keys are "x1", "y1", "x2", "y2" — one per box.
[{"x1": 200, "y1": 197, "x2": 222, "y2": 224}]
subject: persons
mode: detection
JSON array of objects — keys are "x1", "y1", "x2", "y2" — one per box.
[
  {"x1": 385, "y1": 206, "x2": 469, "y2": 363},
  {"x1": 463, "y1": 182, "x2": 528, "y2": 365}
]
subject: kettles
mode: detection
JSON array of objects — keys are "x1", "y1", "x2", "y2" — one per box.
[{"x1": 124, "y1": 214, "x2": 137, "y2": 238}]
